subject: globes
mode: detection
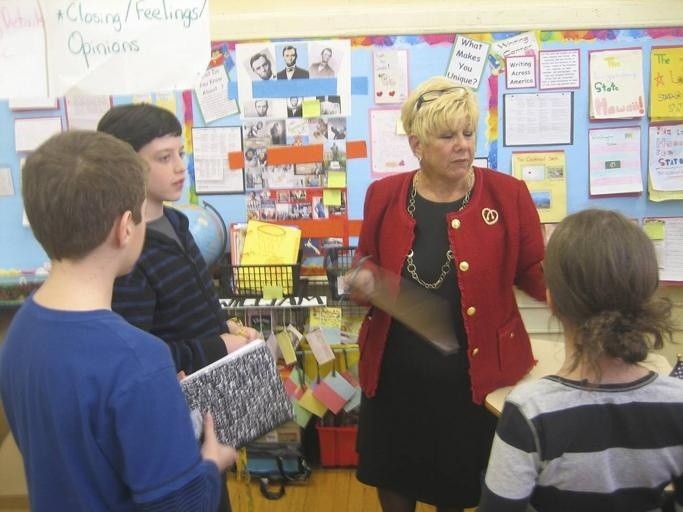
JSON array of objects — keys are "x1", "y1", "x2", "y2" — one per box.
[{"x1": 174, "y1": 201, "x2": 227, "y2": 270}]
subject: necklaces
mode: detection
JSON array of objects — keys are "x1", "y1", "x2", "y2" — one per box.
[{"x1": 407, "y1": 168, "x2": 474, "y2": 290}]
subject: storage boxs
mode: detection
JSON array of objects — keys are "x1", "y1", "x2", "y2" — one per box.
[
  {"x1": 301, "y1": 315, "x2": 360, "y2": 386},
  {"x1": 314, "y1": 420, "x2": 359, "y2": 466}
]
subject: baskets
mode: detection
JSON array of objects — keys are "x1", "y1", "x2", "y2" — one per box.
[
  {"x1": 324, "y1": 247, "x2": 358, "y2": 301},
  {"x1": 221, "y1": 250, "x2": 303, "y2": 297}
]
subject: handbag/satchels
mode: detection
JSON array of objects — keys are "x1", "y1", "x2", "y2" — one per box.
[{"x1": 232, "y1": 442, "x2": 310, "y2": 483}]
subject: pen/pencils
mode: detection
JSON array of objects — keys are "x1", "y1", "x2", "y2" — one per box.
[{"x1": 337, "y1": 265, "x2": 361, "y2": 306}]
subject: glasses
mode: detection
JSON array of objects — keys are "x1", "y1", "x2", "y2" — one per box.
[{"x1": 417, "y1": 87, "x2": 468, "y2": 111}]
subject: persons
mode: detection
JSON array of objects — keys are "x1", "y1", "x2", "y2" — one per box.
[
  {"x1": 96, "y1": 104, "x2": 260, "y2": 511},
  {"x1": 331, "y1": 126, "x2": 345, "y2": 139},
  {"x1": 271, "y1": 122, "x2": 281, "y2": 143},
  {"x1": 255, "y1": 100, "x2": 268, "y2": 116},
  {"x1": 319, "y1": 119, "x2": 328, "y2": 138},
  {"x1": 287, "y1": 97, "x2": 302, "y2": 117},
  {"x1": 248, "y1": 121, "x2": 262, "y2": 137},
  {"x1": 250, "y1": 54, "x2": 275, "y2": 80},
  {"x1": 0, "y1": 130, "x2": 239, "y2": 511},
  {"x1": 307, "y1": 47, "x2": 336, "y2": 75},
  {"x1": 246, "y1": 142, "x2": 340, "y2": 220},
  {"x1": 479, "y1": 210, "x2": 683, "y2": 511},
  {"x1": 277, "y1": 46, "x2": 310, "y2": 79},
  {"x1": 351, "y1": 76, "x2": 549, "y2": 512}
]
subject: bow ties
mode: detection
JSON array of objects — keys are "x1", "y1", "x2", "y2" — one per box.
[{"x1": 287, "y1": 67, "x2": 293, "y2": 70}]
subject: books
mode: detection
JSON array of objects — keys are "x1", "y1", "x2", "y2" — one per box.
[
  {"x1": 184, "y1": 338, "x2": 296, "y2": 449},
  {"x1": 230, "y1": 221, "x2": 302, "y2": 299}
]
